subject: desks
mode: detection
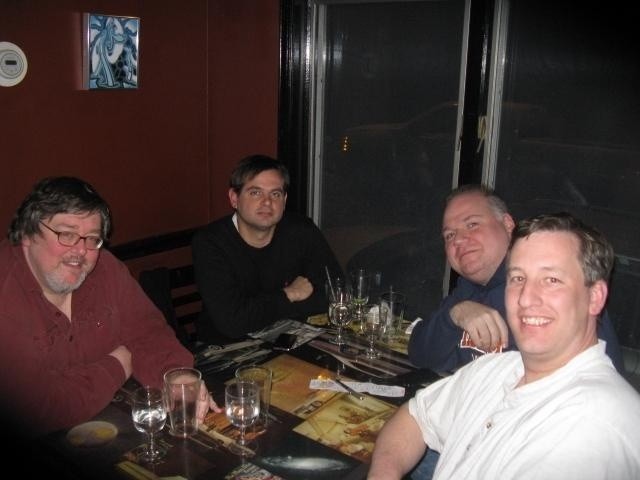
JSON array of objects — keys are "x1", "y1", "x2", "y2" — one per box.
[{"x1": 36, "y1": 305, "x2": 440, "y2": 480}]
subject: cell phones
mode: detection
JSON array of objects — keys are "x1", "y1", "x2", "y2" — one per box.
[
  {"x1": 398, "y1": 368, "x2": 438, "y2": 387},
  {"x1": 272, "y1": 333, "x2": 297, "y2": 352}
]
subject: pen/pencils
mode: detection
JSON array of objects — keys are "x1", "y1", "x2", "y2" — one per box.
[{"x1": 334, "y1": 378, "x2": 365, "y2": 400}]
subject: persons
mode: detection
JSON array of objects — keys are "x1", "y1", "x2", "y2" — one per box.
[
  {"x1": 408, "y1": 185, "x2": 626, "y2": 480},
  {"x1": 366, "y1": 212, "x2": 639, "y2": 480},
  {"x1": 187, "y1": 153, "x2": 345, "y2": 345},
  {"x1": 0, "y1": 174, "x2": 224, "y2": 446}
]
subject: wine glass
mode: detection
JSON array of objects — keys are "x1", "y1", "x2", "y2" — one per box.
[
  {"x1": 358, "y1": 304, "x2": 388, "y2": 360},
  {"x1": 225, "y1": 382, "x2": 260, "y2": 456},
  {"x1": 330, "y1": 293, "x2": 351, "y2": 346},
  {"x1": 131, "y1": 387, "x2": 169, "y2": 463}
]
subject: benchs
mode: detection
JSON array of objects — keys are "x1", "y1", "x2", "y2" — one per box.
[{"x1": 109, "y1": 224, "x2": 208, "y2": 354}]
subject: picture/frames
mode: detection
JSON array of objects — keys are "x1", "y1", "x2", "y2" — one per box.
[{"x1": 82, "y1": 12, "x2": 140, "y2": 91}]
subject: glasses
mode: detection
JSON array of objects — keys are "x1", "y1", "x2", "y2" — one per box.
[{"x1": 39, "y1": 219, "x2": 104, "y2": 251}]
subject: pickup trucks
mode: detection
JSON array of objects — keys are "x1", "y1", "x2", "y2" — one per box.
[{"x1": 342, "y1": 99, "x2": 634, "y2": 203}]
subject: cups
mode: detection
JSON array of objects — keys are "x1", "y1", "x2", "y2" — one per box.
[
  {"x1": 378, "y1": 292, "x2": 407, "y2": 338},
  {"x1": 324, "y1": 277, "x2": 352, "y2": 318},
  {"x1": 235, "y1": 367, "x2": 272, "y2": 430},
  {"x1": 163, "y1": 368, "x2": 201, "y2": 438},
  {"x1": 351, "y1": 269, "x2": 370, "y2": 305}
]
